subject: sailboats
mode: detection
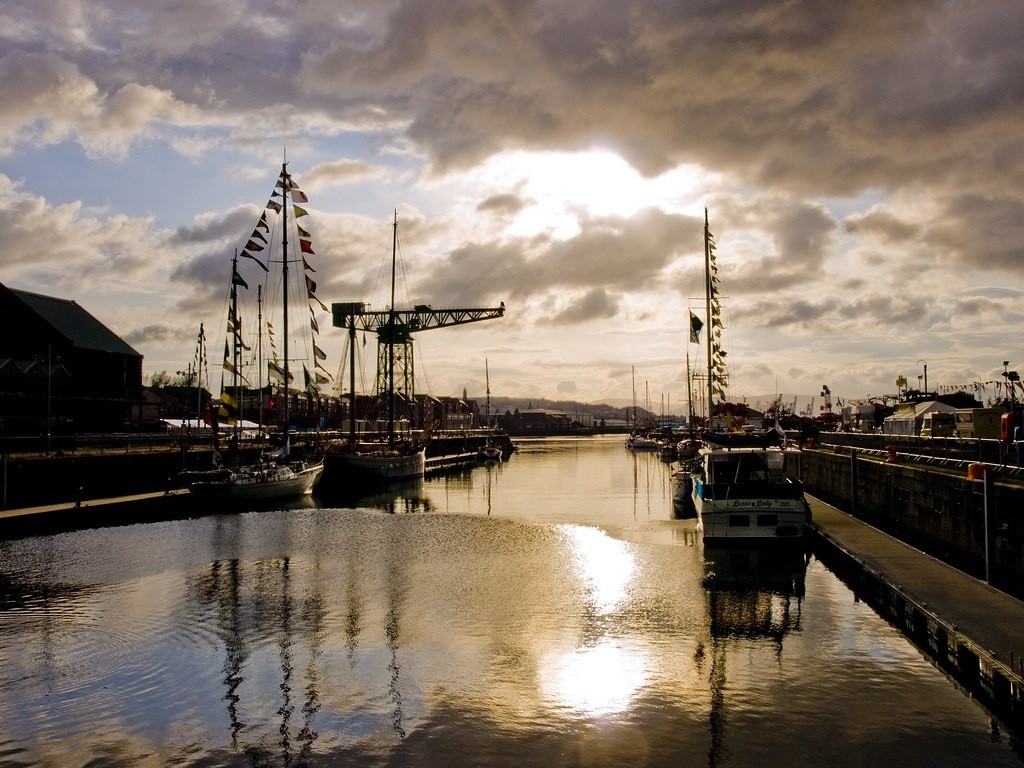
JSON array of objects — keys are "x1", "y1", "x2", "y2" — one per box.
[
  {"x1": 626, "y1": 204, "x2": 816, "y2": 544},
  {"x1": 177, "y1": 149, "x2": 332, "y2": 497},
  {"x1": 323, "y1": 208, "x2": 517, "y2": 481}
]
